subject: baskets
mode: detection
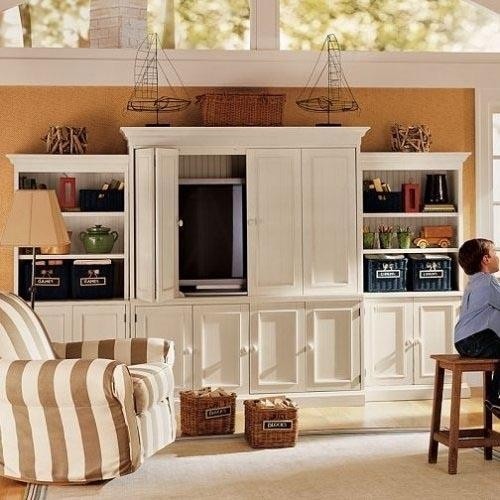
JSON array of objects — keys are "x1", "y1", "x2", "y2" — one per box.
[
  {"x1": 179, "y1": 389, "x2": 237, "y2": 437},
  {"x1": 243, "y1": 400, "x2": 298, "y2": 448},
  {"x1": 194, "y1": 92, "x2": 286, "y2": 127}
]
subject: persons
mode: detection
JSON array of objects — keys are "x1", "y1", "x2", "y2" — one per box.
[{"x1": 454, "y1": 238, "x2": 500, "y2": 419}]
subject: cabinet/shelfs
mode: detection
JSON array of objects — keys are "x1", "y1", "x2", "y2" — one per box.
[
  {"x1": 250, "y1": 303, "x2": 362, "y2": 394},
  {"x1": 361, "y1": 297, "x2": 461, "y2": 389},
  {"x1": 363, "y1": 169, "x2": 461, "y2": 294},
  {"x1": 34, "y1": 304, "x2": 126, "y2": 344},
  {"x1": 134, "y1": 306, "x2": 248, "y2": 396},
  {"x1": 247, "y1": 149, "x2": 359, "y2": 301},
  {"x1": 17, "y1": 170, "x2": 125, "y2": 301}
]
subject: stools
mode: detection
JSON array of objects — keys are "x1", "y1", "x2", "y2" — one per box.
[{"x1": 427, "y1": 353, "x2": 500, "y2": 475}]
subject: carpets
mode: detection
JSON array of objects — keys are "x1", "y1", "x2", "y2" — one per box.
[{"x1": 20, "y1": 433, "x2": 500, "y2": 500}]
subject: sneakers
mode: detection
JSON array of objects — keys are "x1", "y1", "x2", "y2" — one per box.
[{"x1": 484, "y1": 397, "x2": 500, "y2": 418}]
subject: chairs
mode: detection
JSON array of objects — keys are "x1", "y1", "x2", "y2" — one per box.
[{"x1": 0, "y1": 292, "x2": 176, "y2": 484}]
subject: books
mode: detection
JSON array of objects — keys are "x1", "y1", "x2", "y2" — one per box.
[
  {"x1": 21, "y1": 175, "x2": 48, "y2": 190},
  {"x1": 97, "y1": 179, "x2": 124, "y2": 198},
  {"x1": 363, "y1": 176, "x2": 391, "y2": 201}
]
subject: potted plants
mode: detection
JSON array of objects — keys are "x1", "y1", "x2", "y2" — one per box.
[
  {"x1": 377, "y1": 225, "x2": 395, "y2": 248},
  {"x1": 396, "y1": 226, "x2": 411, "y2": 249},
  {"x1": 363, "y1": 225, "x2": 376, "y2": 249}
]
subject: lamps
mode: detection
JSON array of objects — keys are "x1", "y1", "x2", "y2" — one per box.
[{"x1": 0, "y1": 190, "x2": 72, "y2": 309}]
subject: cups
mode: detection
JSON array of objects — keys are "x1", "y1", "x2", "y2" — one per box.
[{"x1": 362, "y1": 232, "x2": 411, "y2": 249}]
